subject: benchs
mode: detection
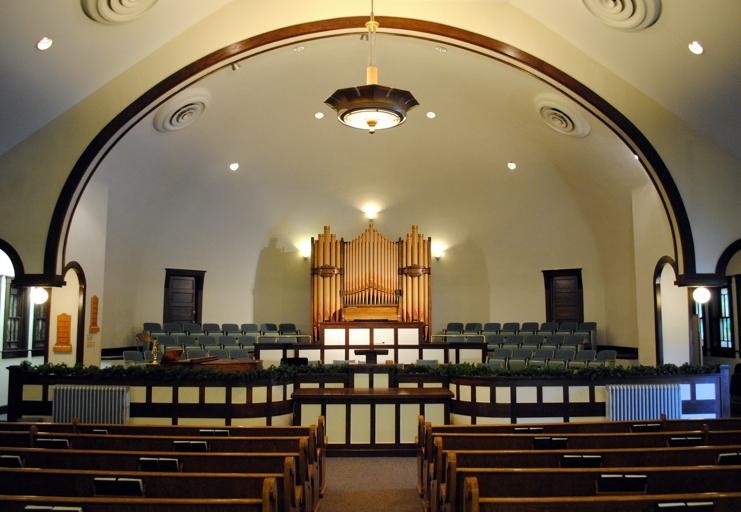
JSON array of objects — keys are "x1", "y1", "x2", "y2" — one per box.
[
  {"x1": 0, "y1": 414, "x2": 329, "y2": 512},
  {"x1": 413, "y1": 413, "x2": 740, "y2": 512}
]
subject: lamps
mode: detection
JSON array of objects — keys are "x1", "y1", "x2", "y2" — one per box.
[{"x1": 322, "y1": 0, "x2": 420, "y2": 130}]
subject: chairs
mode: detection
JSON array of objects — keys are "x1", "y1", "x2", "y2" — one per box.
[
  {"x1": 434, "y1": 322, "x2": 619, "y2": 369},
  {"x1": 122, "y1": 323, "x2": 313, "y2": 364}
]
